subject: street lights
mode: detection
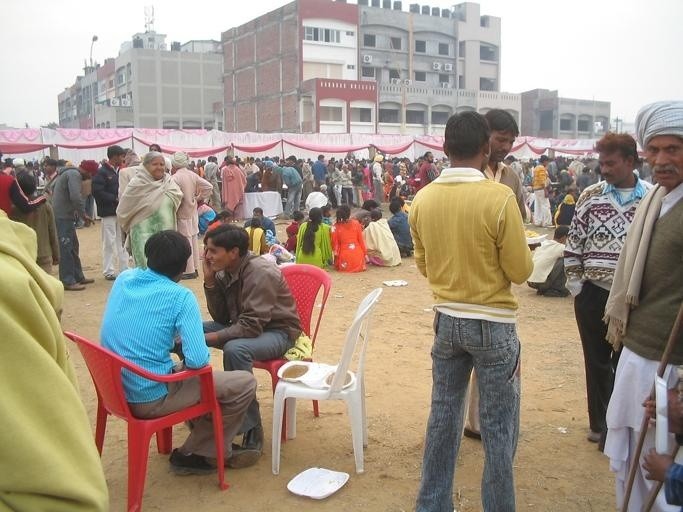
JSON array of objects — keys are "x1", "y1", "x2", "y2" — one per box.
[{"x1": 89, "y1": 34, "x2": 97, "y2": 132}]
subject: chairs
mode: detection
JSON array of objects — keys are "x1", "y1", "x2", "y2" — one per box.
[
  {"x1": 268, "y1": 284, "x2": 384, "y2": 477},
  {"x1": 249, "y1": 262, "x2": 332, "y2": 445},
  {"x1": 61, "y1": 329, "x2": 232, "y2": 512}
]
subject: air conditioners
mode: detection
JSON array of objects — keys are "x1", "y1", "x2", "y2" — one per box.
[
  {"x1": 441, "y1": 82, "x2": 450, "y2": 89},
  {"x1": 391, "y1": 77, "x2": 401, "y2": 85},
  {"x1": 363, "y1": 55, "x2": 372, "y2": 64},
  {"x1": 445, "y1": 63, "x2": 452, "y2": 71},
  {"x1": 432, "y1": 62, "x2": 441, "y2": 70},
  {"x1": 404, "y1": 79, "x2": 412, "y2": 86}
]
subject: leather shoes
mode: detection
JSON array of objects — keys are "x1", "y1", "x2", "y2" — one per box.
[
  {"x1": 242, "y1": 428, "x2": 264, "y2": 451},
  {"x1": 170, "y1": 447, "x2": 217, "y2": 476},
  {"x1": 224, "y1": 443, "x2": 261, "y2": 469}
]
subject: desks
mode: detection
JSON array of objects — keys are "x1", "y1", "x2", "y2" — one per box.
[{"x1": 240, "y1": 191, "x2": 284, "y2": 219}]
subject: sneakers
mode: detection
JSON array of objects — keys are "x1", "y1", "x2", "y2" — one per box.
[
  {"x1": 181, "y1": 269, "x2": 199, "y2": 280},
  {"x1": 105, "y1": 275, "x2": 116, "y2": 280},
  {"x1": 64, "y1": 283, "x2": 86, "y2": 291},
  {"x1": 80, "y1": 279, "x2": 94, "y2": 285},
  {"x1": 537, "y1": 289, "x2": 569, "y2": 296},
  {"x1": 587, "y1": 431, "x2": 601, "y2": 442},
  {"x1": 463, "y1": 427, "x2": 481, "y2": 441}
]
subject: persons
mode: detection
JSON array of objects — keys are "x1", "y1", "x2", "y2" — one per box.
[
  {"x1": 99, "y1": 224, "x2": 301, "y2": 475},
  {"x1": 1, "y1": 153, "x2": 651, "y2": 297},
  {"x1": 406, "y1": 98, "x2": 683, "y2": 511},
  {"x1": 1, "y1": 210, "x2": 111, "y2": 512}
]
